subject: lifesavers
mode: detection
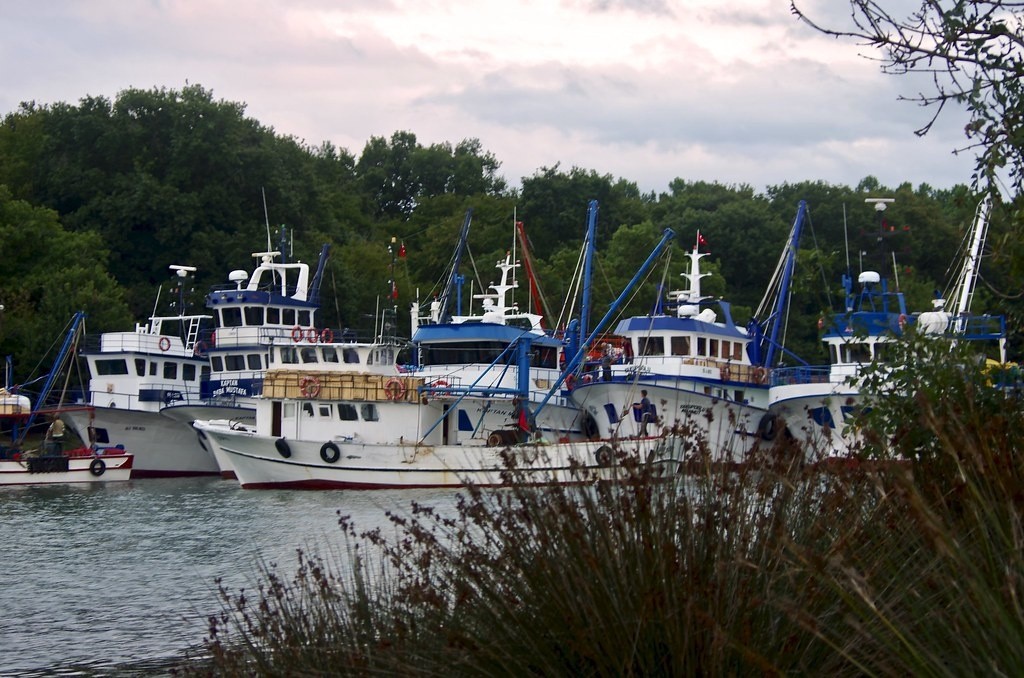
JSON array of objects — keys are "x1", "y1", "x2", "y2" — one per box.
[
  {"x1": 90, "y1": 459, "x2": 106, "y2": 476},
  {"x1": 566, "y1": 373, "x2": 576, "y2": 390},
  {"x1": 755, "y1": 367, "x2": 767, "y2": 384},
  {"x1": 292, "y1": 327, "x2": 303, "y2": 343},
  {"x1": 300, "y1": 376, "x2": 320, "y2": 398},
  {"x1": 758, "y1": 413, "x2": 778, "y2": 441},
  {"x1": 899, "y1": 313, "x2": 906, "y2": 331},
  {"x1": 307, "y1": 327, "x2": 319, "y2": 343},
  {"x1": 319, "y1": 443, "x2": 340, "y2": 464},
  {"x1": 275, "y1": 439, "x2": 291, "y2": 458},
  {"x1": 322, "y1": 328, "x2": 334, "y2": 343},
  {"x1": 720, "y1": 363, "x2": 731, "y2": 381},
  {"x1": 194, "y1": 341, "x2": 209, "y2": 356},
  {"x1": 158, "y1": 337, "x2": 171, "y2": 351},
  {"x1": 594, "y1": 446, "x2": 612, "y2": 465},
  {"x1": 384, "y1": 378, "x2": 405, "y2": 399},
  {"x1": 431, "y1": 380, "x2": 451, "y2": 400}
]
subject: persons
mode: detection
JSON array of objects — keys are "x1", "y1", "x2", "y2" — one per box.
[
  {"x1": 630, "y1": 388, "x2": 652, "y2": 437},
  {"x1": 586, "y1": 342, "x2": 629, "y2": 381},
  {"x1": 48, "y1": 415, "x2": 65, "y2": 454},
  {"x1": 510, "y1": 399, "x2": 537, "y2": 442}
]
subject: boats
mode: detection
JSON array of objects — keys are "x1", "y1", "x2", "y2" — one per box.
[{"x1": 0, "y1": 187, "x2": 1024, "y2": 492}]
